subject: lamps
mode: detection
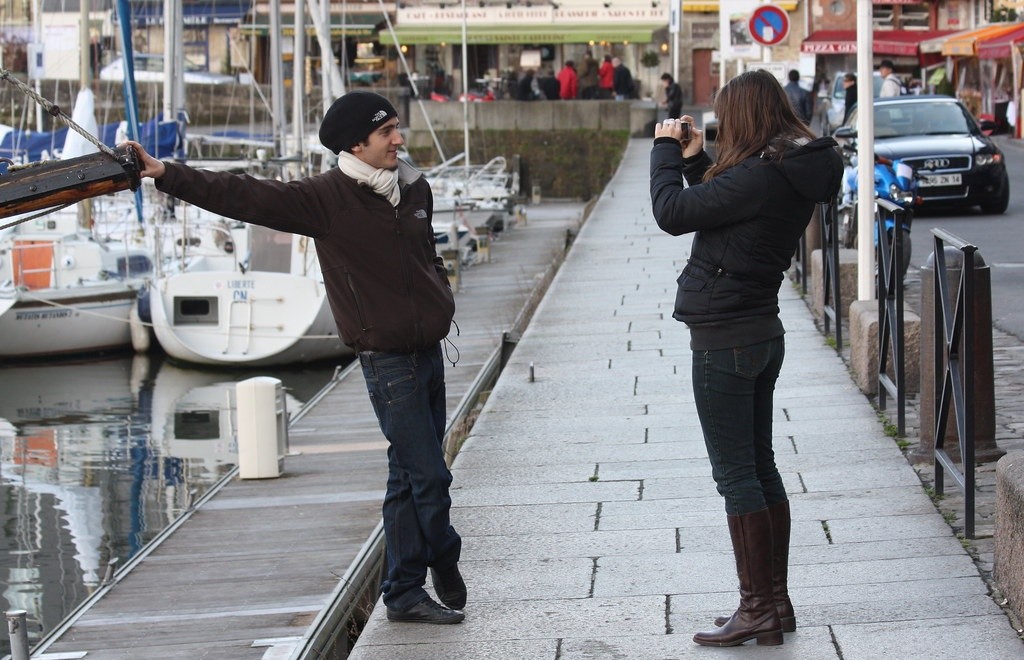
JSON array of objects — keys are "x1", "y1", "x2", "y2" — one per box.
[
  {"x1": 439, "y1": 2, "x2": 453, "y2": 9},
  {"x1": 604, "y1": 1, "x2": 612, "y2": 8},
  {"x1": 549, "y1": 1, "x2": 558, "y2": 9},
  {"x1": 506, "y1": 1, "x2": 512, "y2": 9},
  {"x1": 652, "y1": 1, "x2": 658, "y2": 8},
  {"x1": 400, "y1": 2, "x2": 412, "y2": 9},
  {"x1": 480, "y1": 1, "x2": 493, "y2": 7}
]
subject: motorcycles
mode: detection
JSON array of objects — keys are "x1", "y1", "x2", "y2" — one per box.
[{"x1": 834, "y1": 143, "x2": 925, "y2": 295}]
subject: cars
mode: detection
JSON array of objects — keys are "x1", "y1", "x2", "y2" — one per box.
[
  {"x1": 818, "y1": 71, "x2": 912, "y2": 136},
  {"x1": 98, "y1": 52, "x2": 238, "y2": 86},
  {"x1": 828, "y1": 97, "x2": 1011, "y2": 217}
]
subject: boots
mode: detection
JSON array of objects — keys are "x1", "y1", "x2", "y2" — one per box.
[
  {"x1": 715, "y1": 501, "x2": 798, "y2": 631},
  {"x1": 693, "y1": 507, "x2": 784, "y2": 645}
]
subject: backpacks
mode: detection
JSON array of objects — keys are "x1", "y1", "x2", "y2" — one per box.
[{"x1": 887, "y1": 77, "x2": 909, "y2": 97}]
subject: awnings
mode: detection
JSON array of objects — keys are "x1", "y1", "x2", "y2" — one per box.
[
  {"x1": 109, "y1": 1, "x2": 671, "y2": 44},
  {"x1": 802, "y1": 28, "x2": 961, "y2": 56}
]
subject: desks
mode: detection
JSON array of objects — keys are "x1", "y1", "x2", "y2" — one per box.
[{"x1": 352, "y1": 70, "x2": 383, "y2": 86}]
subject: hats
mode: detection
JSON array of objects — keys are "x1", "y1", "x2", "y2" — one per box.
[
  {"x1": 875, "y1": 60, "x2": 895, "y2": 71},
  {"x1": 319, "y1": 90, "x2": 398, "y2": 155}
]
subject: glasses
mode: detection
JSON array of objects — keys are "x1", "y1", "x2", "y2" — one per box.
[{"x1": 843, "y1": 79, "x2": 852, "y2": 83}]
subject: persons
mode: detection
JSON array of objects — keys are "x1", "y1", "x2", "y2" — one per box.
[
  {"x1": 117, "y1": 91, "x2": 466, "y2": 624},
  {"x1": 499, "y1": 50, "x2": 925, "y2": 127},
  {"x1": 650, "y1": 69, "x2": 843, "y2": 647}
]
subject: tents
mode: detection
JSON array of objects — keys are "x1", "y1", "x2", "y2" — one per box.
[{"x1": 914, "y1": 23, "x2": 1023, "y2": 143}]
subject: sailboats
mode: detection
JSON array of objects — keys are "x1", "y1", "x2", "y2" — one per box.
[
  {"x1": 0, "y1": 0, "x2": 523, "y2": 373},
  {"x1": 0, "y1": 352, "x2": 349, "y2": 641}
]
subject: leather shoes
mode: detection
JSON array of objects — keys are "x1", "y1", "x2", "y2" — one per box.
[
  {"x1": 429, "y1": 562, "x2": 467, "y2": 609},
  {"x1": 386, "y1": 596, "x2": 465, "y2": 624}
]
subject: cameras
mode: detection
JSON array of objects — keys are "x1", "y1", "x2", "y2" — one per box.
[{"x1": 680, "y1": 122, "x2": 692, "y2": 141}]
